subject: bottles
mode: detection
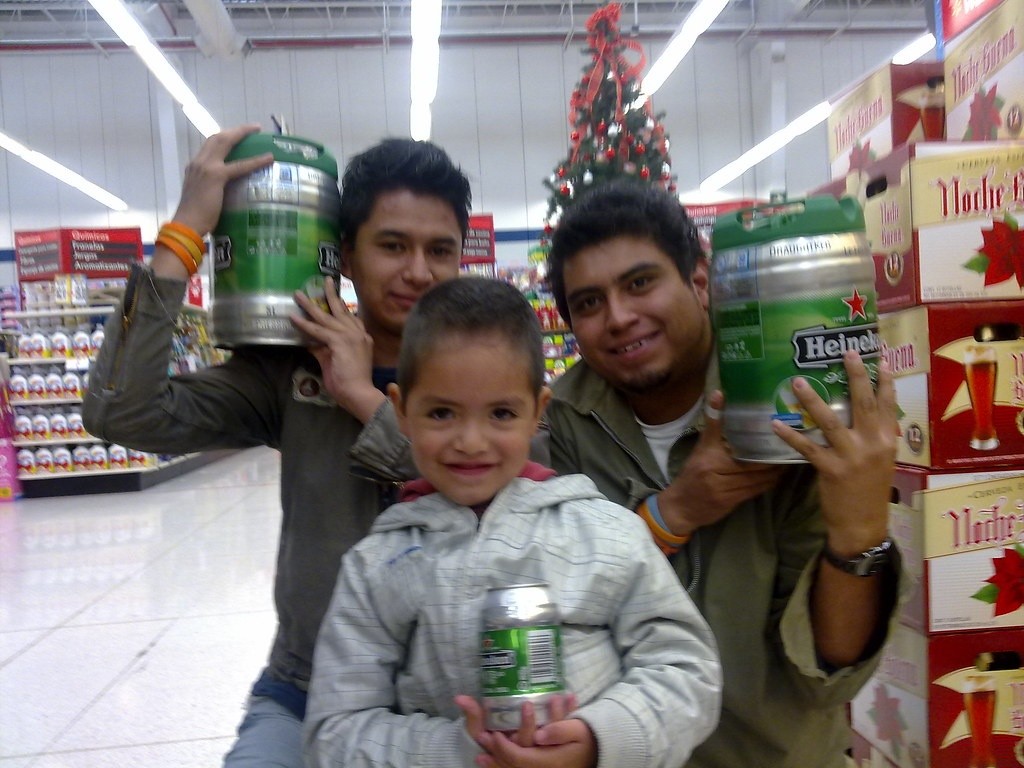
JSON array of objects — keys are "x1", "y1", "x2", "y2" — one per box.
[{"x1": 8, "y1": 322, "x2": 149, "y2": 478}]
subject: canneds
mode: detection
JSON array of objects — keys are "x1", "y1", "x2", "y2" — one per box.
[
  {"x1": 208, "y1": 134, "x2": 344, "y2": 347},
  {"x1": 709, "y1": 193, "x2": 883, "y2": 466},
  {"x1": 480, "y1": 583, "x2": 569, "y2": 731}
]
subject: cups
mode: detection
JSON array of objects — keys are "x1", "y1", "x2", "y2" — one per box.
[
  {"x1": 963, "y1": 677, "x2": 999, "y2": 767},
  {"x1": 964, "y1": 345, "x2": 1000, "y2": 451}
]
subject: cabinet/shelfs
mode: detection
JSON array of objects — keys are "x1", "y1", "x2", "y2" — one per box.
[{"x1": 0, "y1": 308, "x2": 248, "y2": 495}]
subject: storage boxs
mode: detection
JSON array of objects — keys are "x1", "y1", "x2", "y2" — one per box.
[{"x1": 805, "y1": 0, "x2": 1024, "y2": 768}]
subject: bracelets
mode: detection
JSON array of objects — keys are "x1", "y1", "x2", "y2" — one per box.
[
  {"x1": 155, "y1": 222, "x2": 206, "y2": 276},
  {"x1": 823, "y1": 537, "x2": 896, "y2": 579},
  {"x1": 636, "y1": 495, "x2": 690, "y2": 559}
]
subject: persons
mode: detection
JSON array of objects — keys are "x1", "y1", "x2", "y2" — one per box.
[
  {"x1": 298, "y1": 276, "x2": 725, "y2": 768},
  {"x1": 82, "y1": 123, "x2": 554, "y2": 768},
  {"x1": 545, "y1": 177, "x2": 898, "y2": 768}
]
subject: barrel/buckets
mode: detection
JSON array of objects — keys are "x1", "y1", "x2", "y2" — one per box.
[
  {"x1": 706, "y1": 192, "x2": 881, "y2": 465},
  {"x1": 209, "y1": 132, "x2": 344, "y2": 348}
]
